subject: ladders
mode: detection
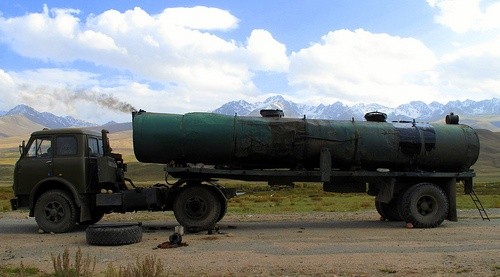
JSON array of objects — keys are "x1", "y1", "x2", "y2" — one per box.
[{"x1": 462, "y1": 181, "x2": 490, "y2": 221}]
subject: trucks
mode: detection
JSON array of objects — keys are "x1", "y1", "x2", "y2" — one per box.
[{"x1": 10, "y1": 110, "x2": 480, "y2": 233}]
